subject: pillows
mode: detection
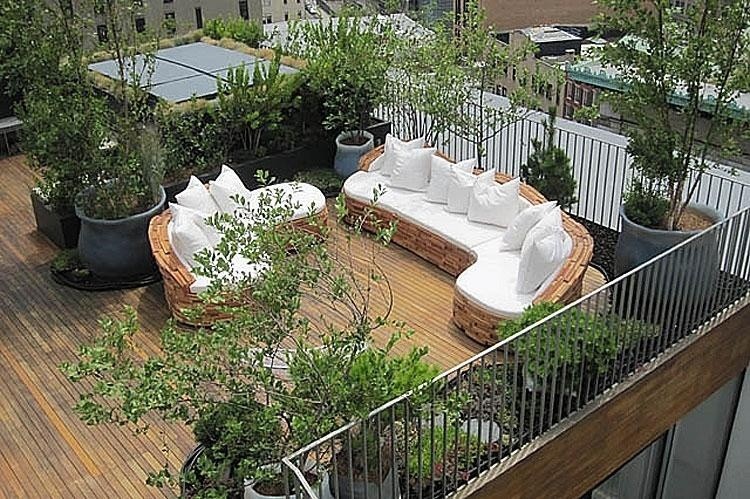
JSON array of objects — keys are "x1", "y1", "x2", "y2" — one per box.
[
  {"x1": 379, "y1": 132, "x2": 525, "y2": 231},
  {"x1": 498, "y1": 197, "x2": 572, "y2": 295},
  {"x1": 168, "y1": 163, "x2": 251, "y2": 283}
]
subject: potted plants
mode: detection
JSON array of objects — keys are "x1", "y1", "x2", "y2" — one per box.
[
  {"x1": 156, "y1": 348, "x2": 439, "y2": 498},
  {"x1": 283, "y1": 4, "x2": 396, "y2": 178},
  {"x1": 590, "y1": 1, "x2": 749, "y2": 328},
  {"x1": 69, "y1": 4, "x2": 169, "y2": 285}
]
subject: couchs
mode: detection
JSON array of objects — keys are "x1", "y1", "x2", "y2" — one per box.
[
  {"x1": 145, "y1": 163, "x2": 329, "y2": 326},
  {"x1": 336, "y1": 133, "x2": 594, "y2": 346}
]
